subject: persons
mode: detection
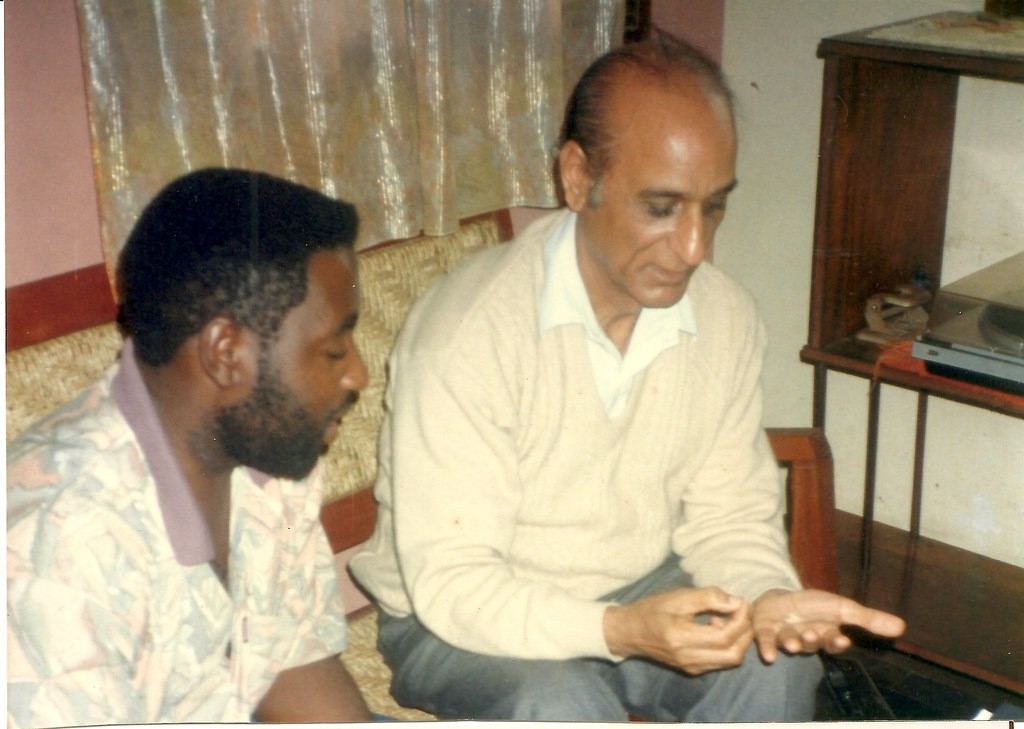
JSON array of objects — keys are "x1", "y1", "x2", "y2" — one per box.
[
  {"x1": 6, "y1": 168, "x2": 377, "y2": 729},
  {"x1": 344, "y1": 36, "x2": 904, "y2": 722}
]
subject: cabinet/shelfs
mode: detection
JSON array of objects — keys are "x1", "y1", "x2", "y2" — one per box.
[{"x1": 798, "y1": 11, "x2": 1024, "y2": 699}]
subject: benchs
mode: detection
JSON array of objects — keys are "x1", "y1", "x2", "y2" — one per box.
[{"x1": 7, "y1": 204, "x2": 838, "y2": 720}]
